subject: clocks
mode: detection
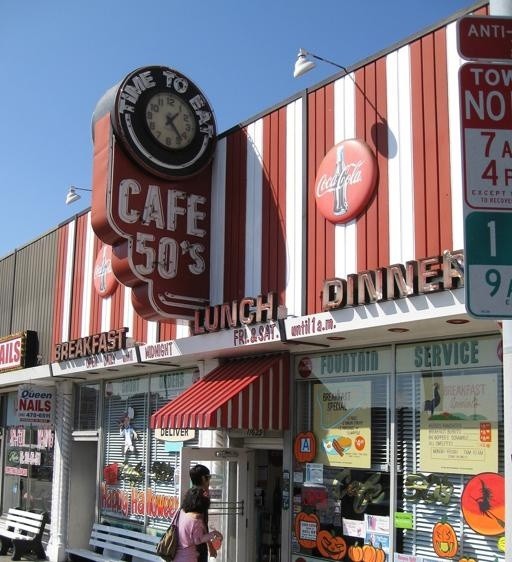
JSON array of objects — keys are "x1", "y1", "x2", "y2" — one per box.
[{"x1": 109, "y1": 65, "x2": 216, "y2": 183}]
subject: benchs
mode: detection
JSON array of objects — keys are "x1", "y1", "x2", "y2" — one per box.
[
  {"x1": 0, "y1": 506, "x2": 49, "y2": 561},
  {"x1": 65, "y1": 520, "x2": 165, "y2": 562}
]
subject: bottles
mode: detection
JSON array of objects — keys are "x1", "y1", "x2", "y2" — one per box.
[
  {"x1": 333, "y1": 147, "x2": 348, "y2": 216},
  {"x1": 99, "y1": 254, "x2": 107, "y2": 291}
]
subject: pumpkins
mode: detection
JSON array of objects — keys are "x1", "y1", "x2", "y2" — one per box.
[
  {"x1": 374, "y1": 542, "x2": 386, "y2": 561},
  {"x1": 361, "y1": 542, "x2": 376, "y2": 562},
  {"x1": 295, "y1": 432, "x2": 315, "y2": 462},
  {"x1": 295, "y1": 510, "x2": 319, "y2": 548},
  {"x1": 349, "y1": 541, "x2": 362, "y2": 561}
]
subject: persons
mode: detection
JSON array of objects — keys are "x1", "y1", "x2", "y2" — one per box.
[
  {"x1": 188, "y1": 464, "x2": 210, "y2": 561},
  {"x1": 170, "y1": 486, "x2": 223, "y2": 562}
]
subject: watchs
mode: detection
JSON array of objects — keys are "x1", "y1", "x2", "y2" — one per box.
[{"x1": 341, "y1": 482, "x2": 356, "y2": 520}]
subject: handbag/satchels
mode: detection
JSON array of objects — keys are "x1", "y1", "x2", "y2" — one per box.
[{"x1": 155, "y1": 525, "x2": 180, "y2": 562}]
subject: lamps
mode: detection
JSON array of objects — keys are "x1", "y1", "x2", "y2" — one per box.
[
  {"x1": 64, "y1": 185, "x2": 92, "y2": 205},
  {"x1": 286, "y1": 46, "x2": 350, "y2": 78}
]
